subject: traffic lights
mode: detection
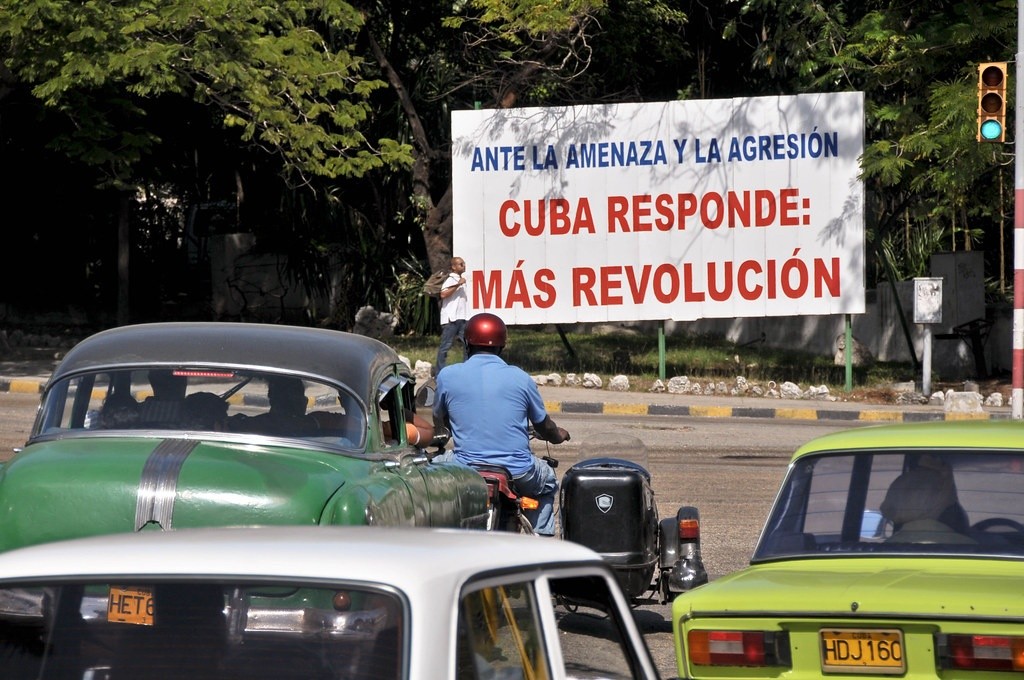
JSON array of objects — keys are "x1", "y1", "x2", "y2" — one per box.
[{"x1": 974, "y1": 63, "x2": 1007, "y2": 145}]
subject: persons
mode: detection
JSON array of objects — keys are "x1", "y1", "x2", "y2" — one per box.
[
  {"x1": 432, "y1": 313, "x2": 571, "y2": 535},
  {"x1": 879, "y1": 469, "x2": 982, "y2": 544},
  {"x1": 435, "y1": 257, "x2": 468, "y2": 378},
  {"x1": 99, "y1": 369, "x2": 435, "y2": 447}
]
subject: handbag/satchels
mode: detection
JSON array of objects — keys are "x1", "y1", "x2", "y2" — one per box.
[{"x1": 423, "y1": 269, "x2": 453, "y2": 295}]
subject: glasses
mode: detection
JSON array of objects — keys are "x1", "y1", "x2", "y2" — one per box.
[{"x1": 456, "y1": 262, "x2": 464, "y2": 267}]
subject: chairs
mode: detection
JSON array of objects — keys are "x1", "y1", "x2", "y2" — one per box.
[
  {"x1": 369, "y1": 624, "x2": 480, "y2": 680},
  {"x1": 873, "y1": 468, "x2": 975, "y2": 548},
  {"x1": 108, "y1": 608, "x2": 232, "y2": 679}
]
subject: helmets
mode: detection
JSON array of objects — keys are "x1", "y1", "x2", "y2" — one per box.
[{"x1": 462, "y1": 313, "x2": 507, "y2": 346}]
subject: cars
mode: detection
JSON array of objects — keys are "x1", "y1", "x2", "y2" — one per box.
[
  {"x1": 667, "y1": 417, "x2": 1023, "y2": 680},
  {"x1": 0, "y1": 322, "x2": 489, "y2": 639},
  {"x1": 1, "y1": 524, "x2": 664, "y2": 679}
]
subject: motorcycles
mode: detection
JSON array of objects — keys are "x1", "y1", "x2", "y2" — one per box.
[{"x1": 440, "y1": 423, "x2": 559, "y2": 534}]
